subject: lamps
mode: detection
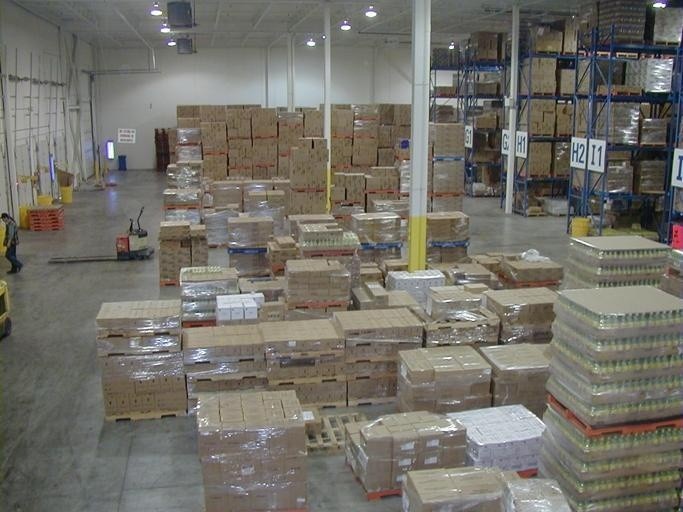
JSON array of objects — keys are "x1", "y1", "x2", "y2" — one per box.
[
  {"x1": 305, "y1": 5, "x2": 377, "y2": 47},
  {"x1": 148, "y1": 1, "x2": 175, "y2": 47}
]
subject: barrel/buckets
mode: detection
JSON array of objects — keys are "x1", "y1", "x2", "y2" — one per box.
[
  {"x1": 572, "y1": 217, "x2": 591, "y2": 238},
  {"x1": 37, "y1": 193, "x2": 53, "y2": 205},
  {"x1": 59, "y1": 185, "x2": 73, "y2": 203},
  {"x1": 19, "y1": 205, "x2": 32, "y2": 230}
]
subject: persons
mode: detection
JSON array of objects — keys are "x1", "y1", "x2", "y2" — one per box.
[{"x1": 0, "y1": 213, "x2": 22, "y2": 275}]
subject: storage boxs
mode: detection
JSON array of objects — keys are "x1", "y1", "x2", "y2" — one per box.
[
  {"x1": 400, "y1": 236, "x2": 683, "y2": 512},
  {"x1": 157, "y1": 103, "x2": 471, "y2": 512},
  {"x1": 576, "y1": 101, "x2": 668, "y2": 193},
  {"x1": 472, "y1": 105, "x2": 503, "y2": 132},
  {"x1": 96, "y1": 299, "x2": 189, "y2": 416},
  {"x1": 517, "y1": 98, "x2": 574, "y2": 179},
  {"x1": 430, "y1": 1, "x2": 683, "y2": 102}
]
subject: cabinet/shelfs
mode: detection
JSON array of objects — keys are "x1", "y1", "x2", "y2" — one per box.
[
  {"x1": 456, "y1": 47, "x2": 503, "y2": 196},
  {"x1": 567, "y1": 25, "x2": 682, "y2": 237},
  {"x1": 500, "y1": 37, "x2": 574, "y2": 217}
]
